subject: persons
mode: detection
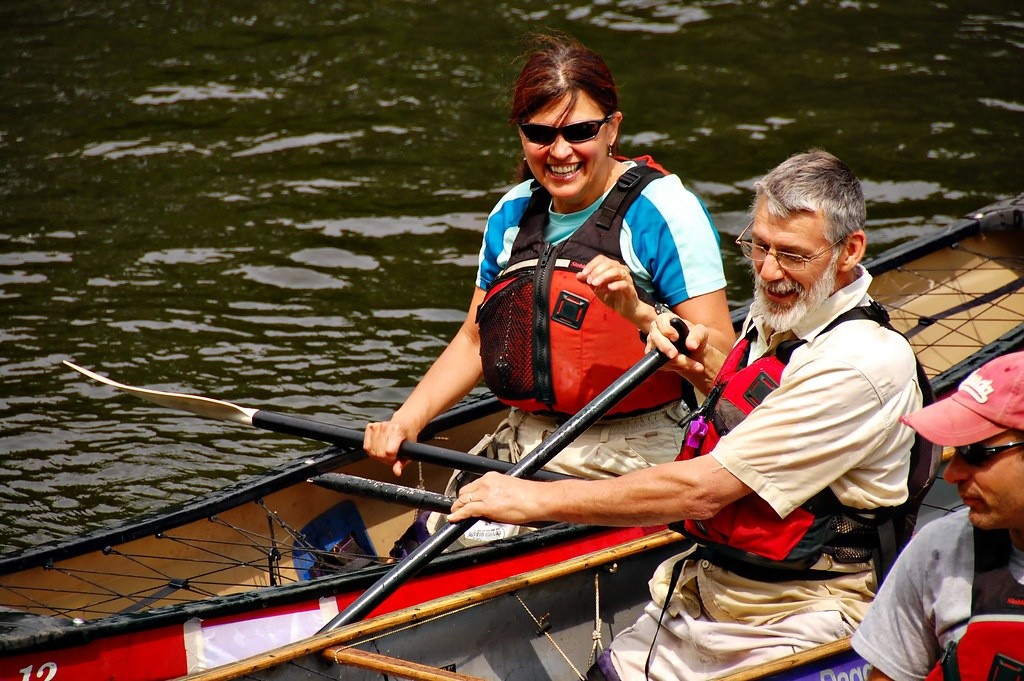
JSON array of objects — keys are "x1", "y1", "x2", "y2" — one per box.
[
  {"x1": 851, "y1": 349, "x2": 1024, "y2": 681},
  {"x1": 443, "y1": 146, "x2": 930, "y2": 681},
  {"x1": 363, "y1": 32, "x2": 740, "y2": 566}
]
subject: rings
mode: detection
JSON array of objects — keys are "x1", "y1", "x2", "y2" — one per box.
[{"x1": 468, "y1": 492, "x2": 473, "y2": 501}]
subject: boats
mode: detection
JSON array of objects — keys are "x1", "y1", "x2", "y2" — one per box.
[{"x1": 0, "y1": 191, "x2": 1023, "y2": 681}]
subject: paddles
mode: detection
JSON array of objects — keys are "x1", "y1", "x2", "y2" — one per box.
[
  {"x1": 303, "y1": 313, "x2": 701, "y2": 640},
  {"x1": 59, "y1": 356, "x2": 598, "y2": 489}
]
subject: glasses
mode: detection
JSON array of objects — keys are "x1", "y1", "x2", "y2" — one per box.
[
  {"x1": 736, "y1": 218, "x2": 847, "y2": 271},
  {"x1": 954, "y1": 441, "x2": 1024, "y2": 465},
  {"x1": 517, "y1": 114, "x2": 612, "y2": 145}
]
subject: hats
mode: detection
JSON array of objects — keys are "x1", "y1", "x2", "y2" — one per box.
[{"x1": 898, "y1": 351, "x2": 1024, "y2": 446}]
